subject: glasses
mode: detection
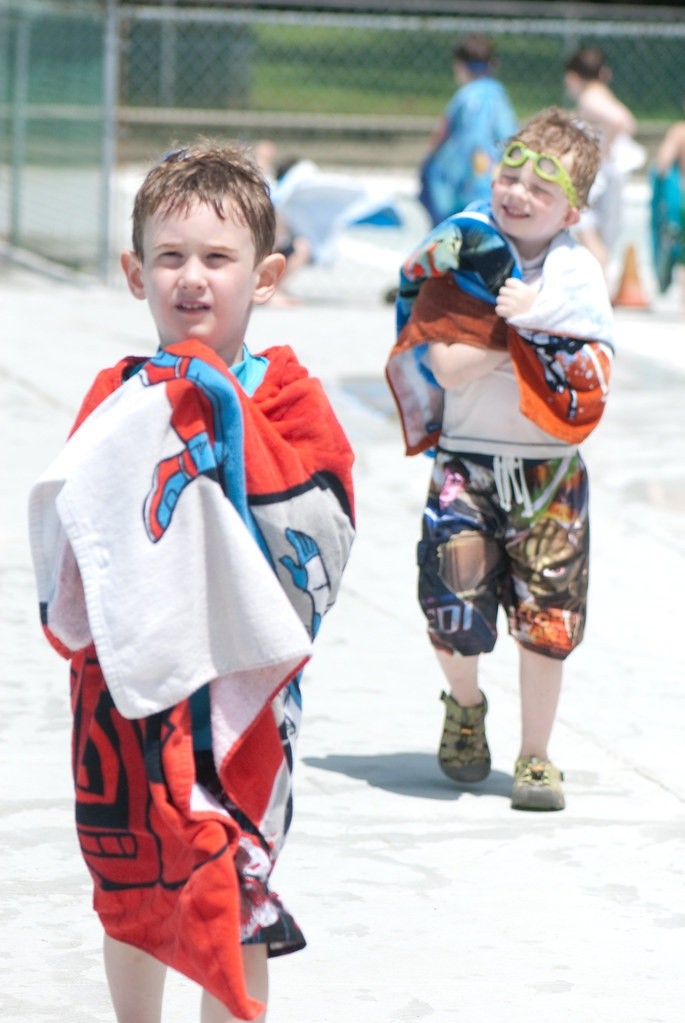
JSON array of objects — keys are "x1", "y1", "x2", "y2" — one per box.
[{"x1": 502, "y1": 140, "x2": 578, "y2": 210}]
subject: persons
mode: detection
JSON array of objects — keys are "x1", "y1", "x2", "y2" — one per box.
[
  {"x1": 563, "y1": 45, "x2": 637, "y2": 265},
  {"x1": 433, "y1": 35, "x2": 518, "y2": 144},
  {"x1": 26, "y1": 146, "x2": 358, "y2": 1023},
  {"x1": 653, "y1": 120, "x2": 685, "y2": 314},
  {"x1": 384, "y1": 105, "x2": 616, "y2": 810}
]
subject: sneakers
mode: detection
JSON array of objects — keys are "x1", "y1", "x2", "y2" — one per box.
[
  {"x1": 439, "y1": 690, "x2": 492, "y2": 781},
  {"x1": 511, "y1": 756, "x2": 565, "y2": 810}
]
